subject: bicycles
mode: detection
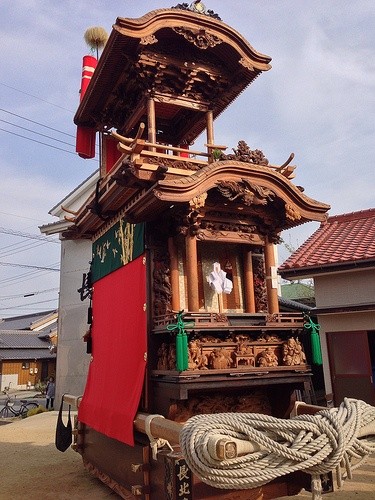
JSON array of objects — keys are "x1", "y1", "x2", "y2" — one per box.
[{"x1": 0, "y1": 394, "x2": 39, "y2": 418}]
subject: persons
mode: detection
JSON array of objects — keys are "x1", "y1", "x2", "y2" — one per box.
[
  {"x1": 152, "y1": 334, "x2": 308, "y2": 370},
  {"x1": 44, "y1": 376, "x2": 55, "y2": 410}
]
